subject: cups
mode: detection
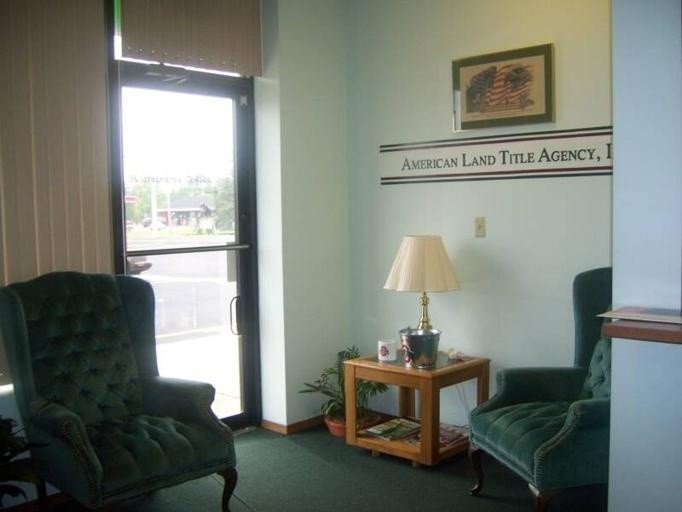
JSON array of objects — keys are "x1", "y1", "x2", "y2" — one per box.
[{"x1": 377, "y1": 340, "x2": 397, "y2": 362}]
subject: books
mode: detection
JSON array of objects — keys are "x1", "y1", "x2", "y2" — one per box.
[{"x1": 365, "y1": 418, "x2": 469, "y2": 447}]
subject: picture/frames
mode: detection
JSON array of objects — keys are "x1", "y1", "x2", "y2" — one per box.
[{"x1": 451, "y1": 44, "x2": 557, "y2": 135}]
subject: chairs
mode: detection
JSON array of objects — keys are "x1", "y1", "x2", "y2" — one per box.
[
  {"x1": 465, "y1": 265, "x2": 611, "y2": 511},
  {"x1": 3, "y1": 272, "x2": 238, "y2": 509}
]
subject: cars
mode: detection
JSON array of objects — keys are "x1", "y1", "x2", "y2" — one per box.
[{"x1": 124, "y1": 216, "x2": 174, "y2": 233}]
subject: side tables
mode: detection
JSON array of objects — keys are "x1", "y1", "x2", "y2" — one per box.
[{"x1": 344, "y1": 354, "x2": 490, "y2": 465}]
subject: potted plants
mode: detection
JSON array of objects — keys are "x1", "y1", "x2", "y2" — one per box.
[{"x1": 295, "y1": 348, "x2": 382, "y2": 439}]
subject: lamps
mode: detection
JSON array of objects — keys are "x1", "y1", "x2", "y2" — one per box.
[{"x1": 379, "y1": 234, "x2": 463, "y2": 366}]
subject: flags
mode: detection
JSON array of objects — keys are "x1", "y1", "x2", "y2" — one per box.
[{"x1": 470, "y1": 64, "x2": 533, "y2": 112}]
